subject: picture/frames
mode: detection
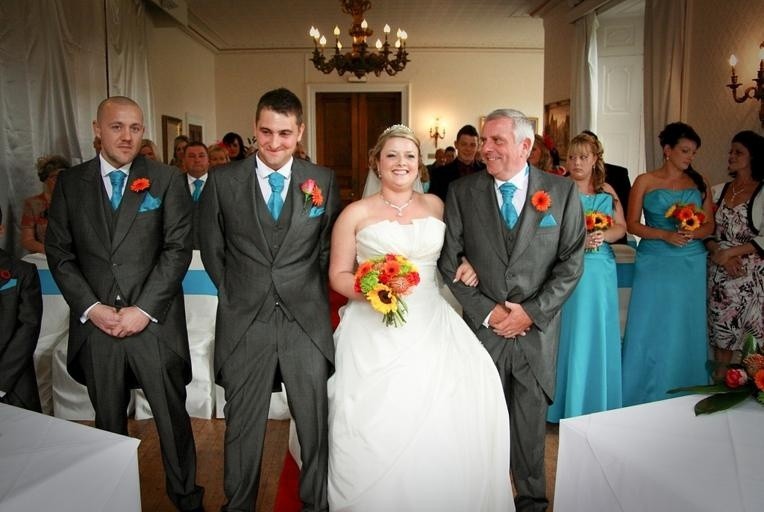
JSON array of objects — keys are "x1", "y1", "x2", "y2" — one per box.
[
  {"x1": 185, "y1": 111, "x2": 207, "y2": 145},
  {"x1": 160, "y1": 115, "x2": 182, "y2": 165}
]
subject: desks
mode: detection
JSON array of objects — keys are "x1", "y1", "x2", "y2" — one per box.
[
  {"x1": 1, "y1": 399, "x2": 143, "y2": 512},
  {"x1": 552, "y1": 392, "x2": 764, "y2": 511}
]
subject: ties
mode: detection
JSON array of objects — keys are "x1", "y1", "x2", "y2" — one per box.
[
  {"x1": 268, "y1": 172, "x2": 283, "y2": 220},
  {"x1": 500, "y1": 183, "x2": 518, "y2": 228},
  {"x1": 192, "y1": 180, "x2": 202, "y2": 201},
  {"x1": 108, "y1": 171, "x2": 125, "y2": 209}
]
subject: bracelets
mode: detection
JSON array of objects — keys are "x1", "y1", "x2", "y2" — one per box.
[{"x1": 714, "y1": 246, "x2": 721, "y2": 255}]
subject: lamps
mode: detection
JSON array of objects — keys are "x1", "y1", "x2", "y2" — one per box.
[
  {"x1": 725, "y1": 43, "x2": 763, "y2": 136},
  {"x1": 431, "y1": 116, "x2": 448, "y2": 148},
  {"x1": 307, "y1": 0, "x2": 409, "y2": 80}
]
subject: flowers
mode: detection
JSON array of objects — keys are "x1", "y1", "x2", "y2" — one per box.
[
  {"x1": 301, "y1": 179, "x2": 323, "y2": 209},
  {"x1": 584, "y1": 208, "x2": 615, "y2": 253},
  {"x1": 353, "y1": 252, "x2": 421, "y2": 327},
  {"x1": 667, "y1": 333, "x2": 764, "y2": 418},
  {"x1": 130, "y1": 178, "x2": 150, "y2": 194},
  {"x1": 531, "y1": 190, "x2": 552, "y2": 210},
  {"x1": 664, "y1": 201, "x2": 707, "y2": 244}
]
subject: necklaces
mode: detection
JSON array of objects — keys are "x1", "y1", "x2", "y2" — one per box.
[
  {"x1": 730, "y1": 183, "x2": 744, "y2": 202},
  {"x1": 379, "y1": 192, "x2": 416, "y2": 217}
]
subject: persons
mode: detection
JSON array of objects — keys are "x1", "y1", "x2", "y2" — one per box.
[
  {"x1": 44, "y1": 95, "x2": 206, "y2": 512},
  {"x1": 621, "y1": 122, "x2": 716, "y2": 407},
  {"x1": 426, "y1": 124, "x2": 633, "y2": 244},
  {"x1": 193, "y1": 89, "x2": 342, "y2": 512},
  {"x1": 546, "y1": 132, "x2": 628, "y2": 424},
  {"x1": 327, "y1": 123, "x2": 517, "y2": 512},
  {"x1": 436, "y1": 109, "x2": 586, "y2": 512},
  {"x1": 138, "y1": 131, "x2": 312, "y2": 251},
  {"x1": 20, "y1": 152, "x2": 72, "y2": 253},
  {"x1": 700, "y1": 130, "x2": 763, "y2": 386},
  {"x1": 0, "y1": 208, "x2": 42, "y2": 414}
]
